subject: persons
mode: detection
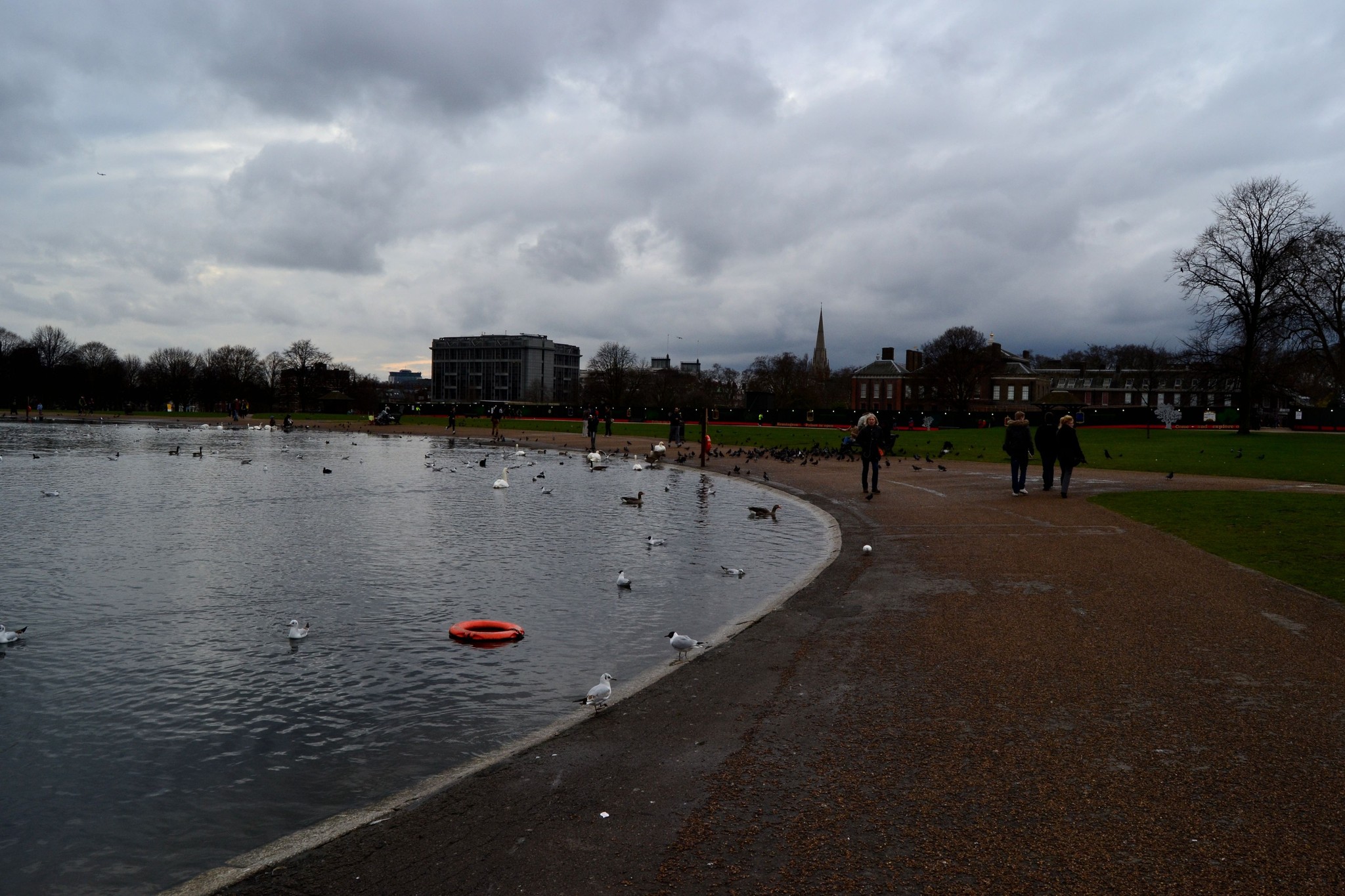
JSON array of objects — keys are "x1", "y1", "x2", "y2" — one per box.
[
  {"x1": 11, "y1": 396, "x2": 521, "y2": 436},
  {"x1": 758, "y1": 414, "x2": 763, "y2": 426},
  {"x1": 909, "y1": 418, "x2": 913, "y2": 430},
  {"x1": 1035, "y1": 412, "x2": 1058, "y2": 491},
  {"x1": 1273, "y1": 415, "x2": 1279, "y2": 428},
  {"x1": 588, "y1": 412, "x2": 599, "y2": 451},
  {"x1": 603, "y1": 406, "x2": 613, "y2": 437},
  {"x1": 668, "y1": 408, "x2": 681, "y2": 447},
  {"x1": 1003, "y1": 411, "x2": 1034, "y2": 496},
  {"x1": 977, "y1": 417, "x2": 995, "y2": 429},
  {"x1": 1056, "y1": 415, "x2": 1084, "y2": 498},
  {"x1": 855, "y1": 415, "x2": 884, "y2": 493},
  {"x1": 893, "y1": 417, "x2": 898, "y2": 431},
  {"x1": 837, "y1": 421, "x2": 858, "y2": 449},
  {"x1": 582, "y1": 407, "x2": 589, "y2": 437}
]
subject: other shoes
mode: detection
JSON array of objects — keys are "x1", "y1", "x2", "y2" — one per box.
[
  {"x1": 864, "y1": 490, "x2": 869, "y2": 494},
  {"x1": 602, "y1": 435, "x2": 607, "y2": 437},
  {"x1": 668, "y1": 443, "x2": 670, "y2": 447},
  {"x1": 453, "y1": 431, "x2": 455, "y2": 435},
  {"x1": 446, "y1": 427, "x2": 448, "y2": 432},
  {"x1": 676, "y1": 443, "x2": 681, "y2": 447},
  {"x1": 872, "y1": 489, "x2": 880, "y2": 493},
  {"x1": 607, "y1": 435, "x2": 612, "y2": 437}
]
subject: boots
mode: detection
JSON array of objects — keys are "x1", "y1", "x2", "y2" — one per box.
[{"x1": 590, "y1": 440, "x2": 596, "y2": 451}]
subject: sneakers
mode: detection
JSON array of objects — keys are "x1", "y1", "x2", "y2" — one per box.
[
  {"x1": 1012, "y1": 491, "x2": 1018, "y2": 496},
  {"x1": 1019, "y1": 487, "x2": 1028, "y2": 494}
]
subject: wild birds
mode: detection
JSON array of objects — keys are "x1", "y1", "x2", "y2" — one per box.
[
  {"x1": 0, "y1": 625, "x2": 30, "y2": 643},
  {"x1": 40, "y1": 490, "x2": 61, "y2": 496},
  {"x1": 572, "y1": 673, "x2": 617, "y2": 715},
  {"x1": 0, "y1": 415, "x2": 1264, "y2": 689}
]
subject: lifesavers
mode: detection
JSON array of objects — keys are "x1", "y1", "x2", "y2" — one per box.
[
  {"x1": 705, "y1": 435, "x2": 711, "y2": 451},
  {"x1": 449, "y1": 620, "x2": 524, "y2": 639}
]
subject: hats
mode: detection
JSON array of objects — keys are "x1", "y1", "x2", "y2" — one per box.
[
  {"x1": 1044, "y1": 412, "x2": 1054, "y2": 424},
  {"x1": 270, "y1": 416, "x2": 274, "y2": 419}
]
